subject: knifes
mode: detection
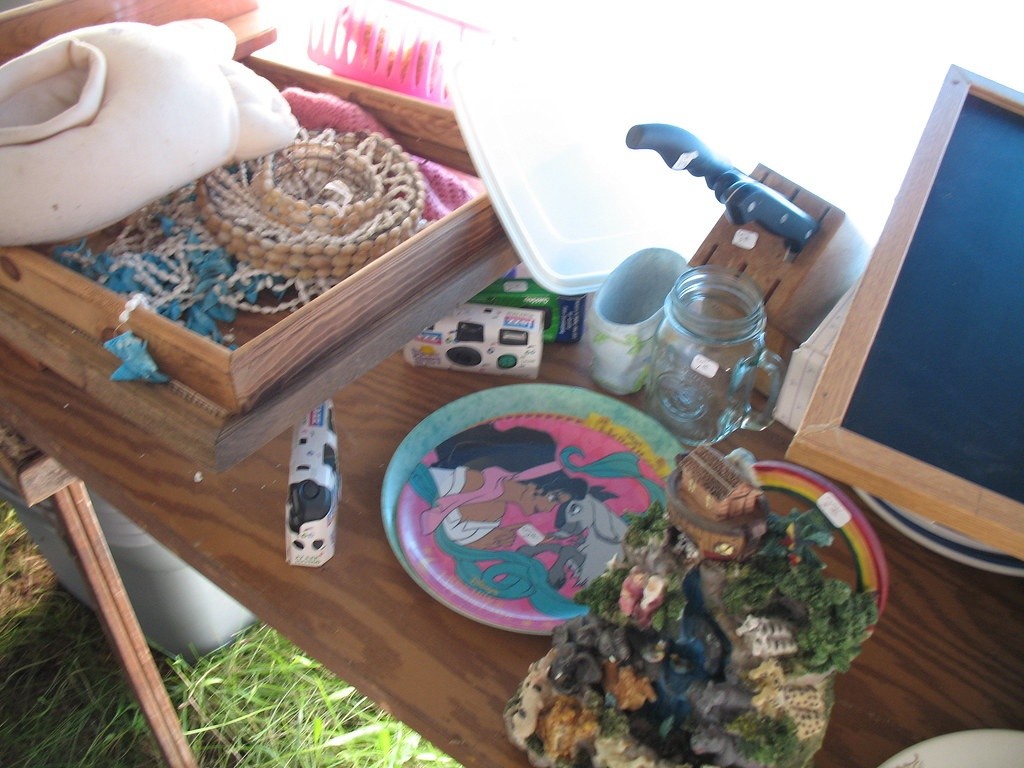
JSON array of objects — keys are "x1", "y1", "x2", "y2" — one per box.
[{"x1": 625, "y1": 123, "x2": 818, "y2": 264}]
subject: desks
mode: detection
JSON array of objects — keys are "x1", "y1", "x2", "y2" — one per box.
[{"x1": 0, "y1": 49, "x2": 1020, "y2": 766}]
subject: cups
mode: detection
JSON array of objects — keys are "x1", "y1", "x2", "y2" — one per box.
[{"x1": 644, "y1": 264, "x2": 785, "y2": 446}]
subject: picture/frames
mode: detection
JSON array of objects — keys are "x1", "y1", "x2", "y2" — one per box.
[{"x1": 784, "y1": 63, "x2": 1023, "y2": 560}]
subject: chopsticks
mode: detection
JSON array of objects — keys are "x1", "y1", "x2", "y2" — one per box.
[{"x1": 56, "y1": 478, "x2": 199, "y2": 768}]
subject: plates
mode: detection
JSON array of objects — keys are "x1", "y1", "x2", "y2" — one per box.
[
  {"x1": 379, "y1": 381, "x2": 684, "y2": 638},
  {"x1": 748, "y1": 458, "x2": 889, "y2": 629},
  {"x1": 875, "y1": 728, "x2": 1024, "y2": 768}
]
